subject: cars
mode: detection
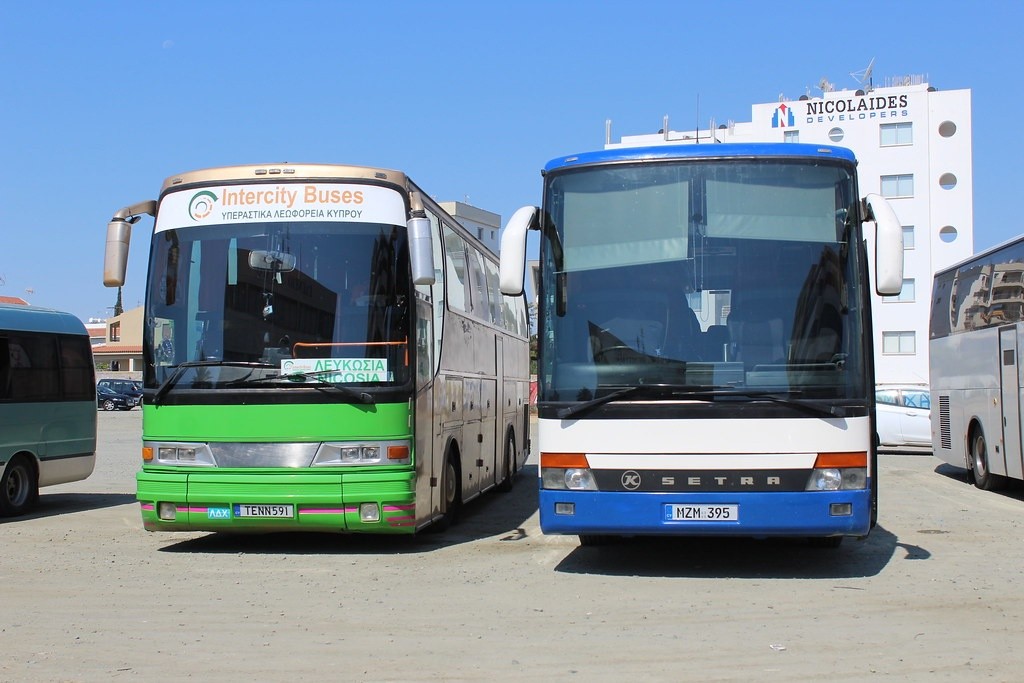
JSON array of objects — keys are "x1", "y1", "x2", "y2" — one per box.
[
  {"x1": 133, "y1": 381, "x2": 143, "y2": 390},
  {"x1": 96, "y1": 385, "x2": 135, "y2": 410},
  {"x1": 96, "y1": 378, "x2": 143, "y2": 408},
  {"x1": 0, "y1": 304, "x2": 97, "y2": 518}
]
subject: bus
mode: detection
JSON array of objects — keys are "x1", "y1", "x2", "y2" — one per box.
[
  {"x1": 929, "y1": 233, "x2": 1024, "y2": 489},
  {"x1": 497, "y1": 142, "x2": 904, "y2": 545},
  {"x1": 102, "y1": 162, "x2": 532, "y2": 535}
]
